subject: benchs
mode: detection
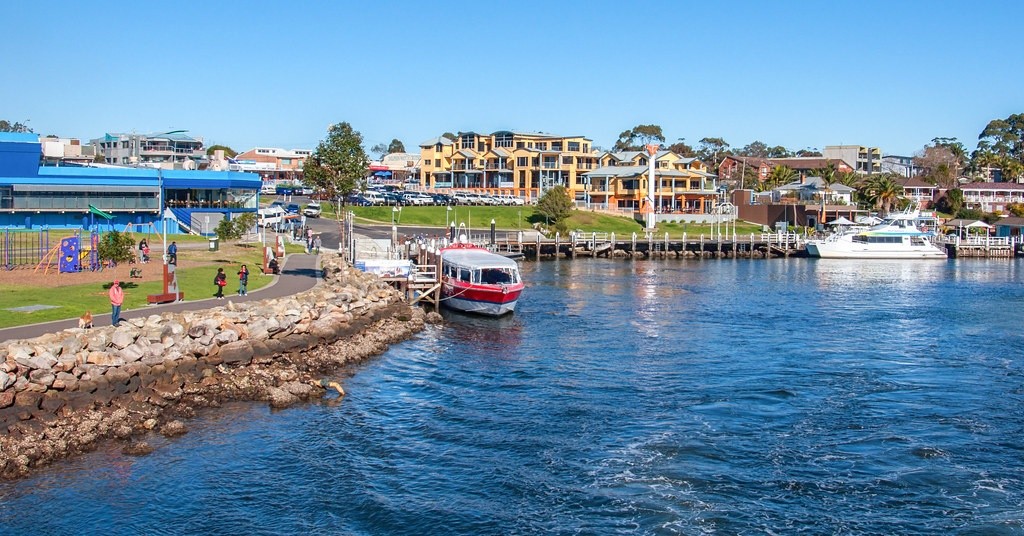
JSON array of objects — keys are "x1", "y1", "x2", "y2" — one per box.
[
  {"x1": 147, "y1": 292, "x2": 184, "y2": 305},
  {"x1": 265, "y1": 268, "x2": 273, "y2": 275},
  {"x1": 277, "y1": 251, "x2": 284, "y2": 258}
]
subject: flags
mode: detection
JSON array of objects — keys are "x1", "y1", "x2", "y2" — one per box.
[{"x1": 90, "y1": 204, "x2": 118, "y2": 219}]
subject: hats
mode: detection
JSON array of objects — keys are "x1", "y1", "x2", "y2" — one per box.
[{"x1": 114, "y1": 279, "x2": 119, "y2": 283}]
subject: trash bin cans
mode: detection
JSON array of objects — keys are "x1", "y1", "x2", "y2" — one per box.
[{"x1": 208, "y1": 238, "x2": 219, "y2": 251}]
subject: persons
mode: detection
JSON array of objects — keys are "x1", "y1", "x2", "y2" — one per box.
[
  {"x1": 214, "y1": 268, "x2": 226, "y2": 297},
  {"x1": 294, "y1": 224, "x2": 322, "y2": 254},
  {"x1": 109, "y1": 280, "x2": 124, "y2": 328},
  {"x1": 400, "y1": 233, "x2": 438, "y2": 246},
  {"x1": 142, "y1": 244, "x2": 150, "y2": 263},
  {"x1": 237, "y1": 264, "x2": 249, "y2": 297},
  {"x1": 168, "y1": 241, "x2": 177, "y2": 266},
  {"x1": 139, "y1": 238, "x2": 148, "y2": 264}
]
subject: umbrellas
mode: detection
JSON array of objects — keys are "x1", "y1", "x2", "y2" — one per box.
[
  {"x1": 858, "y1": 216, "x2": 881, "y2": 226},
  {"x1": 826, "y1": 216, "x2": 857, "y2": 226}
]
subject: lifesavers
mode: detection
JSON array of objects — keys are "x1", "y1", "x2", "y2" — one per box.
[{"x1": 922, "y1": 226, "x2": 928, "y2": 233}]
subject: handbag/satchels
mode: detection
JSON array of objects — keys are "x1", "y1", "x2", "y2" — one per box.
[{"x1": 220, "y1": 281, "x2": 227, "y2": 286}]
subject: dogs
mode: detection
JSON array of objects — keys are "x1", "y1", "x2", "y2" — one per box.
[{"x1": 78, "y1": 310, "x2": 94, "y2": 329}]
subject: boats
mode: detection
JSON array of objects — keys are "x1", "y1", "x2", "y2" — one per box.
[
  {"x1": 428, "y1": 241, "x2": 526, "y2": 318},
  {"x1": 805, "y1": 219, "x2": 949, "y2": 259}
]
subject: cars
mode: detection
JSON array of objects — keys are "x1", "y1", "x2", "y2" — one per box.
[
  {"x1": 346, "y1": 184, "x2": 525, "y2": 207},
  {"x1": 269, "y1": 200, "x2": 285, "y2": 213},
  {"x1": 277, "y1": 187, "x2": 303, "y2": 196},
  {"x1": 285, "y1": 204, "x2": 301, "y2": 215}
]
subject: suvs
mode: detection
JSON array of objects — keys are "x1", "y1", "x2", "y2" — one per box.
[{"x1": 303, "y1": 203, "x2": 322, "y2": 217}]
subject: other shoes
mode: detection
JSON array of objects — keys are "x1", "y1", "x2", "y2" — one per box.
[
  {"x1": 116, "y1": 322, "x2": 122, "y2": 326},
  {"x1": 113, "y1": 324, "x2": 119, "y2": 327}
]
